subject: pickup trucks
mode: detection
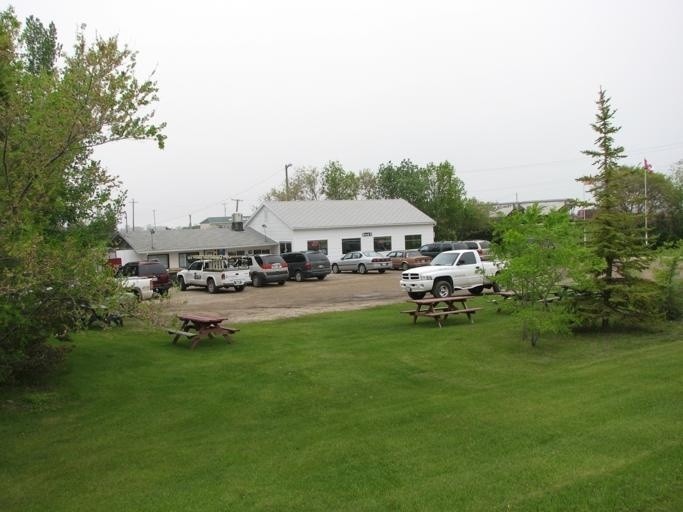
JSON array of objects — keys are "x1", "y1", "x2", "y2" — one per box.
[
  {"x1": 120, "y1": 276, "x2": 154, "y2": 304},
  {"x1": 177, "y1": 259, "x2": 252, "y2": 294},
  {"x1": 400, "y1": 250, "x2": 511, "y2": 298}
]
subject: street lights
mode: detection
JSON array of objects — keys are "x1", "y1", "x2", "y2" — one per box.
[{"x1": 285, "y1": 164, "x2": 292, "y2": 201}]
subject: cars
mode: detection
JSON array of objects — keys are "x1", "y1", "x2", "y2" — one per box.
[
  {"x1": 332, "y1": 240, "x2": 490, "y2": 274},
  {"x1": 115, "y1": 260, "x2": 173, "y2": 297},
  {"x1": 247, "y1": 251, "x2": 330, "y2": 287}
]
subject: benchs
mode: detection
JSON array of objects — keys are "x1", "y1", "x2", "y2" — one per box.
[
  {"x1": 400, "y1": 282, "x2": 584, "y2": 328},
  {"x1": 80, "y1": 304, "x2": 240, "y2": 351}
]
